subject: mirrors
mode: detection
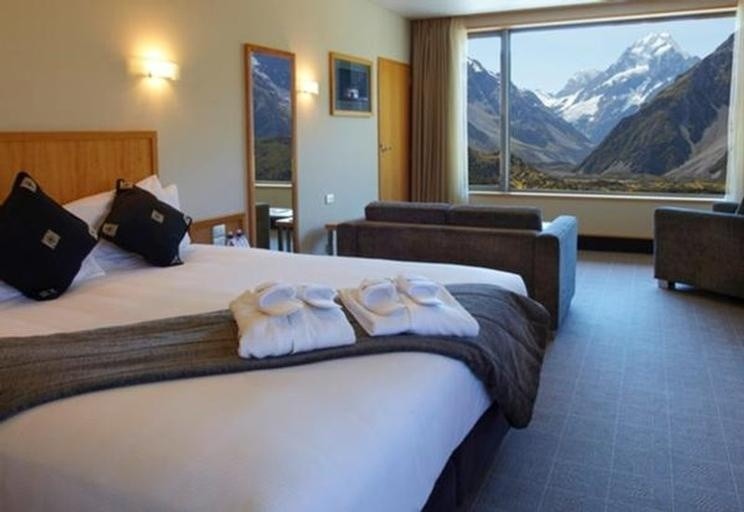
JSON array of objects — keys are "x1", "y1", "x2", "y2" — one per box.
[{"x1": 243, "y1": 43, "x2": 301, "y2": 253}]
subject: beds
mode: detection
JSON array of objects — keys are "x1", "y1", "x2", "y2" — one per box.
[{"x1": 2, "y1": 132, "x2": 528, "y2": 512}]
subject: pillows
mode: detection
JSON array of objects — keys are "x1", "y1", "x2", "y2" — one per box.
[{"x1": 2, "y1": 168, "x2": 194, "y2": 304}]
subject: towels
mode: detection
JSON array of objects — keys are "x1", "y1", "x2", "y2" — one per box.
[{"x1": 228, "y1": 271, "x2": 482, "y2": 362}]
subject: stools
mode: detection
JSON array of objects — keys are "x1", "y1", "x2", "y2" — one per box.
[{"x1": 268, "y1": 205, "x2": 292, "y2": 252}]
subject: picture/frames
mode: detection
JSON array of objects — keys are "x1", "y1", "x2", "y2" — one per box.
[{"x1": 329, "y1": 49, "x2": 376, "y2": 120}]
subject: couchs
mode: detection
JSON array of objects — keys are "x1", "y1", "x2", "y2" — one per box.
[{"x1": 325, "y1": 198, "x2": 581, "y2": 335}]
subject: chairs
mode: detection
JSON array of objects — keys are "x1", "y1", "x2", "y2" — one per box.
[{"x1": 651, "y1": 197, "x2": 744, "y2": 301}]
